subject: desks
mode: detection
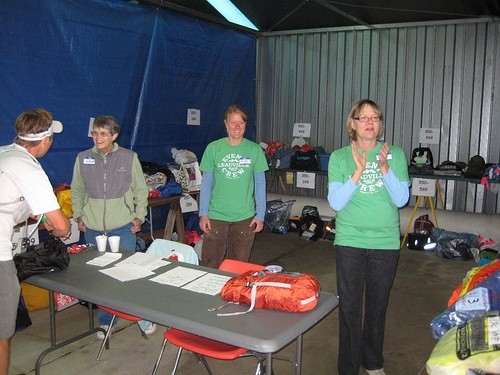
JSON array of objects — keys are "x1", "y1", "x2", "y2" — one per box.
[
  {"x1": 147, "y1": 190, "x2": 200, "y2": 243},
  {"x1": 23, "y1": 243, "x2": 341, "y2": 375}
]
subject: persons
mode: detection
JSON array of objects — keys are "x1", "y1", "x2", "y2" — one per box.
[
  {"x1": 71, "y1": 115, "x2": 156, "y2": 341},
  {"x1": 198, "y1": 105, "x2": 269, "y2": 272},
  {"x1": 0, "y1": 108, "x2": 72, "y2": 375},
  {"x1": 325, "y1": 99, "x2": 410, "y2": 375}
]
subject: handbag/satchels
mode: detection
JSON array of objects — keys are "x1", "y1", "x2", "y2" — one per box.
[
  {"x1": 15, "y1": 295, "x2": 32, "y2": 331},
  {"x1": 140, "y1": 147, "x2": 203, "y2": 199},
  {"x1": 52, "y1": 182, "x2": 74, "y2": 219},
  {"x1": 259, "y1": 138, "x2": 326, "y2": 172},
  {"x1": 262, "y1": 198, "x2": 336, "y2": 242},
  {"x1": 13, "y1": 234, "x2": 70, "y2": 283},
  {"x1": 421, "y1": 259, "x2": 500, "y2": 375},
  {"x1": 406, "y1": 214, "x2": 500, "y2": 266},
  {"x1": 134, "y1": 234, "x2": 152, "y2": 252},
  {"x1": 435, "y1": 154, "x2": 499, "y2": 191}
]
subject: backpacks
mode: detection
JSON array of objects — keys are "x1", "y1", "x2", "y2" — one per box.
[
  {"x1": 179, "y1": 191, "x2": 203, "y2": 262},
  {"x1": 409, "y1": 142, "x2": 436, "y2": 176},
  {"x1": 207, "y1": 271, "x2": 321, "y2": 316}
]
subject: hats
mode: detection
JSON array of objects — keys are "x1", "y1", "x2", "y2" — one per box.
[{"x1": 17, "y1": 119, "x2": 63, "y2": 142}]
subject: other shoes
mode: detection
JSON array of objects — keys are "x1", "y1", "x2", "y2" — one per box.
[
  {"x1": 144, "y1": 321, "x2": 157, "y2": 335},
  {"x1": 97, "y1": 325, "x2": 113, "y2": 339}
]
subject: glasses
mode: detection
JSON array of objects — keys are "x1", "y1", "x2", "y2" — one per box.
[
  {"x1": 353, "y1": 116, "x2": 381, "y2": 122},
  {"x1": 91, "y1": 130, "x2": 111, "y2": 136}
]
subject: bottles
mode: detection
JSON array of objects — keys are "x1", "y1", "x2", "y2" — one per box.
[{"x1": 167, "y1": 249, "x2": 184, "y2": 262}]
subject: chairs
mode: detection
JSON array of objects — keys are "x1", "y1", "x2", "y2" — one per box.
[
  {"x1": 163, "y1": 259, "x2": 271, "y2": 375},
  {"x1": 97, "y1": 238, "x2": 201, "y2": 375}
]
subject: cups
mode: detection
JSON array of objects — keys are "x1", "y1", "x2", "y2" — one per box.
[
  {"x1": 95, "y1": 235, "x2": 108, "y2": 252},
  {"x1": 108, "y1": 236, "x2": 121, "y2": 252},
  {"x1": 266, "y1": 265, "x2": 283, "y2": 273}
]
